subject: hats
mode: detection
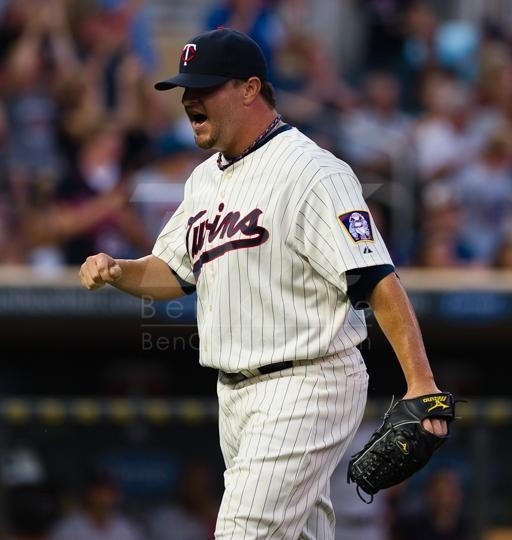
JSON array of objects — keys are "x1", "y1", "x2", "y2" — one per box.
[{"x1": 155, "y1": 27, "x2": 267, "y2": 90}]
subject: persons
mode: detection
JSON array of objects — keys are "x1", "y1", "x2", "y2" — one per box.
[{"x1": 0, "y1": 0, "x2": 512, "y2": 540}]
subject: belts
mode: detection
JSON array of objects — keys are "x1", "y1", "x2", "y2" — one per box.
[{"x1": 217, "y1": 361, "x2": 293, "y2": 385}]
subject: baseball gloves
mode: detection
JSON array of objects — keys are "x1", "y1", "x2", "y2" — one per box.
[{"x1": 347, "y1": 392, "x2": 467, "y2": 503}]
